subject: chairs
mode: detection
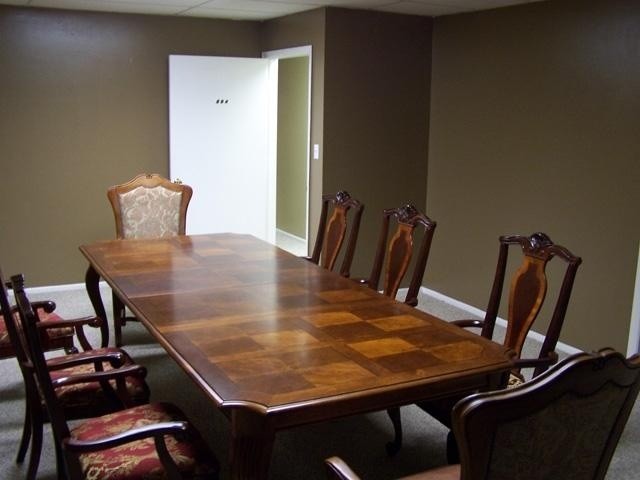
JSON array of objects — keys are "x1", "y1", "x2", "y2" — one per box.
[
  {"x1": 387, "y1": 233, "x2": 583, "y2": 454},
  {"x1": 349, "y1": 200, "x2": 438, "y2": 306},
  {"x1": 1, "y1": 307, "x2": 80, "y2": 361},
  {"x1": 298, "y1": 191, "x2": 365, "y2": 275},
  {"x1": 107, "y1": 172, "x2": 193, "y2": 348},
  {"x1": 2, "y1": 280, "x2": 150, "y2": 480},
  {"x1": 9, "y1": 273, "x2": 212, "y2": 479},
  {"x1": 331, "y1": 347, "x2": 639, "y2": 479}
]
